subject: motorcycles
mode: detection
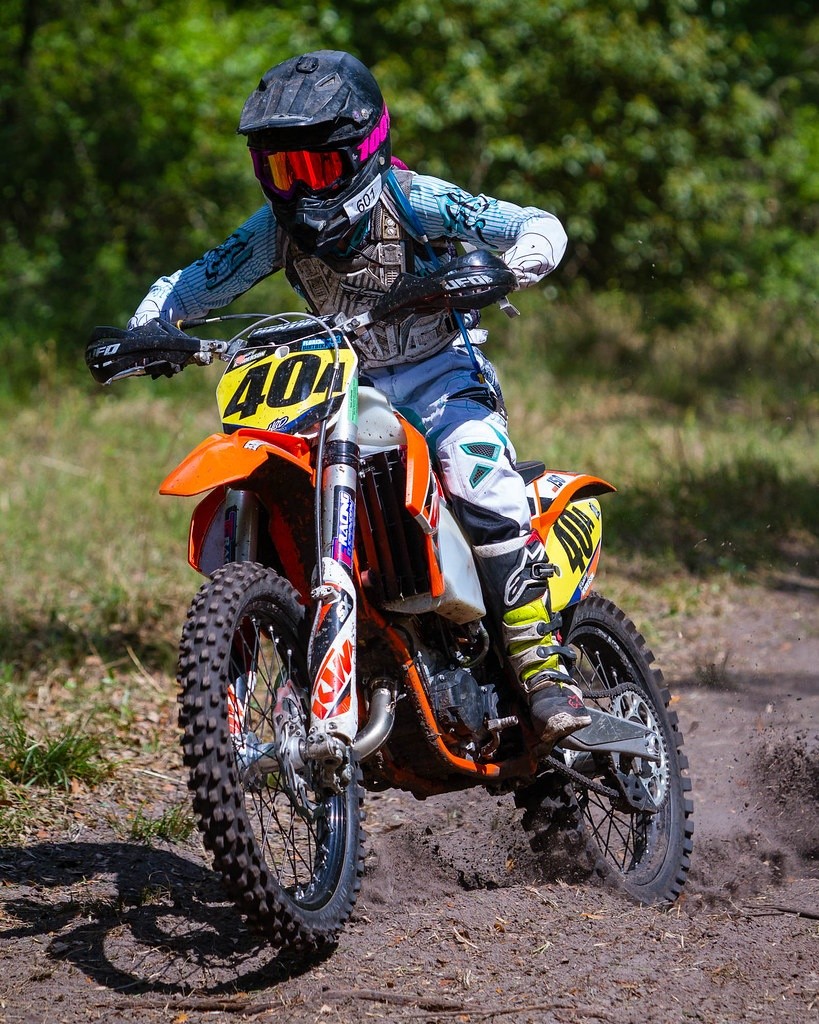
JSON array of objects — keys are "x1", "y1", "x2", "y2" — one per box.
[{"x1": 83, "y1": 267, "x2": 696, "y2": 956}]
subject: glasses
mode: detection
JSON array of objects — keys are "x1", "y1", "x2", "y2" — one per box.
[{"x1": 247, "y1": 137, "x2": 362, "y2": 201}]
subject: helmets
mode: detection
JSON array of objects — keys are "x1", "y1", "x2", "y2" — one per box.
[{"x1": 236, "y1": 49, "x2": 392, "y2": 258}]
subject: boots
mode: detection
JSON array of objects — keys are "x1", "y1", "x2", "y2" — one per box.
[{"x1": 469, "y1": 528, "x2": 593, "y2": 733}]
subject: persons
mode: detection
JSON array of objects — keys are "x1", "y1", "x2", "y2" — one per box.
[{"x1": 127, "y1": 50, "x2": 593, "y2": 743}]
